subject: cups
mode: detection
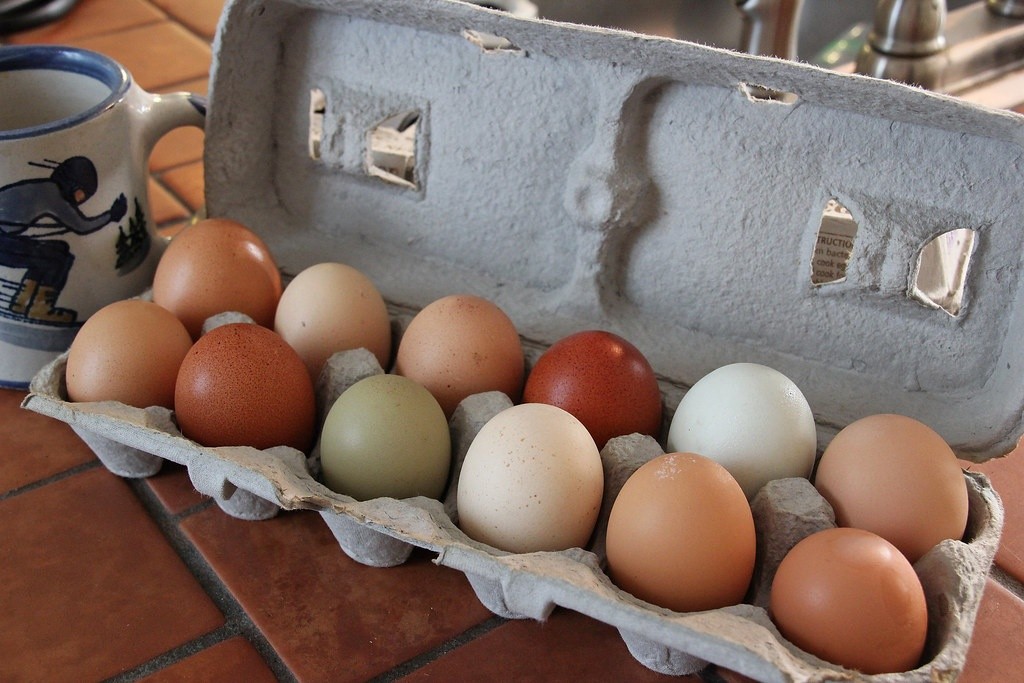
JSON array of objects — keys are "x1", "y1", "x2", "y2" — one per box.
[{"x1": 1, "y1": 45, "x2": 207, "y2": 391}]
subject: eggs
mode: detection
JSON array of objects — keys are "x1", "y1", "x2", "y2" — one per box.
[{"x1": 64, "y1": 217, "x2": 976, "y2": 674}]
